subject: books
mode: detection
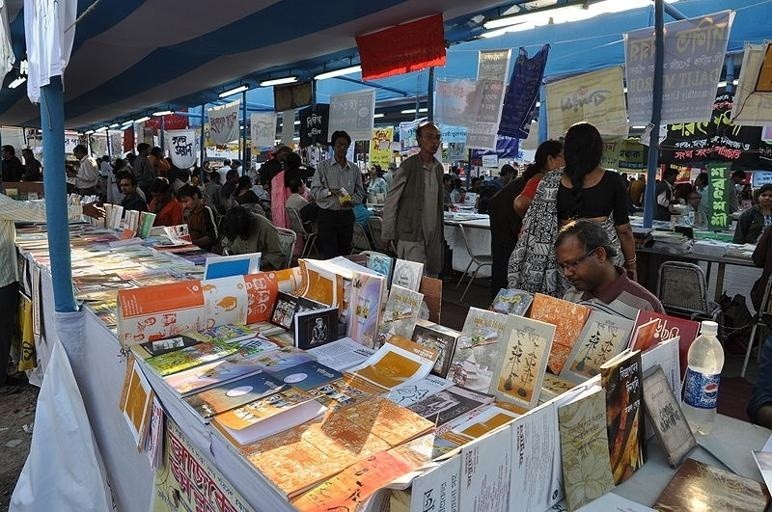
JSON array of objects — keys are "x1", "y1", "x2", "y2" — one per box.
[
  {"x1": 528, "y1": 291, "x2": 589, "y2": 375},
  {"x1": 446, "y1": 307, "x2": 509, "y2": 403},
  {"x1": 643, "y1": 365, "x2": 699, "y2": 467},
  {"x1": 115, "y1": 324, "x2": 456, "y2": 512},
  {"x1": 107, "y1": 208, "x2": 207, "y2": 327},
  {"x1": 558, "y1": 386, "x2": 616, "y2": 512},
  {"x1": 490, "y1": 313, "x2": 557, "y2": 410},
  {"x1": 207, "y1": 254, "x2": 424, "y2": 329},
  {"x1": 652, "y1": 458, "x2": 771, "y2": 511},
  {"x1": 600, "y1": 348, "x2": 648, "y2": 486},
  {"x1": 559, "y1": 311, "x2": 636, "y2": 386},
  {"x1": 444, "y1": 384, "x2": 526, "y2": 447},
  {"x1": 638, "y1": 224, "x2": 753, "y2": 257},
  {"x1": 14, "y1": 221, "x2": 106, "y2": 315}
]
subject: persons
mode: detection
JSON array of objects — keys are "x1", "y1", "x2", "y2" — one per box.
[
  {"x1": 148, "y1": 177, "x2": 183, "y2": 228},
  {"x1": 146, "y1": 147, "x2": 171, "y2": 176},
  {"x1": 729, "y1": 171, "x2": 746, "y2": 214},
  {"x1": 134, "y1": 142, "x2": 154, "y2": 204},
  {"x1": 177, "y1": 184, "x2": 219, "y2": 253},
  {"x1": 513, "y1": 139, "x2": 566, "y2": 219},
  {"x1": 631, "y1": 174, "x2": 646, "y2": 207},
  {"x1": 202, "y1": 162, "x2": 210, "y2": 184},
  {"x1": 285, "y1": 179, "x2": 311, "y2": 233},
  {"x1": 485, "y1": 164, "x2": 514, "y2": 216},
  {"x1": 221, "y1": 170, "x2": 239, "y2": 200},
  {"x1": 107, "y1": 158, "x2": 126, "y2": 206},
  {"x1": 383, "y1": 162, "x2": 396, "y2": 187},
  {"x1": 681, "y1": 191, "x2": 708, "y2": 232},
  {"x1": 219, "y1": 206, "x2": 285, "y2": 272},
  {"x1": 234, "y1": 176, "x2": 260, "y2": 205},
  {"x1": 22, "y1": 148, "x2": 43, "y2": 182},
  {"x1": 439, "y1": 165, "x2": 488, "y2": 214},
  {"x1": 101, "y1": 155, "x2": 113, "y2": 192},
  {"x1": 67, "y1": 145, "x2": 98, "y2": 195},
  {"x1": 230, "y1": 160, "x2": 243, "y2": 177},
  {"x1": 746, "y1": 225, "x2": 771, "y2": 320},
  {"x1": 271, "y1": 152, "x2": 310, "y2": 230},
  {"x1": 1, "y1": 194, "x2": 106, "y2": 386},
  {"x1": 508, "y1": 124, "x2": 636, "y2": 300},
  {"x1": 217, "y1": 159, "x2": 232, "y2": 185},
  {"x1": 367, "y1": 164, "x2": 387, "y2": 208},
  {"x1": 693, "y1": 172, "x2": 709, "y2": 213},
  {"x1": 733, "y1": 184, "x2": 771, "y2": 244},
  {"x1": 310, "y1": 131, "x2": 365, "y2": 260},
  {"x1": 119, "y1": 174, "x2": 150, "y2": 220},
  {"x1": 554, "y1": 217, "x2": 665, "y2": 320},
  {"x1": 381, "y1": 123, "x2": 444, "y2": 277},
  {"x1": 1, "y1": 145, "x2": 22, "y2": 182},
  {"x1": 654, "y1": 168, "x2": 679, "y2": 222},
  {"x1": 621, "y1": 173, "x2": 631, "y2": 203}
]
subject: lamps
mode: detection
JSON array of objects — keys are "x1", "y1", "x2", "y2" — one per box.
[
  {"x1": 482, "y1": 3, "x2": 588, "y2": 29},
  {"x1": 85, "y1": 130, "x2": 94, "y2": 134},
  {"x1": 109, "y1": 123, "x2": 121, "y2": 128},
  {"x1": 77, "y1": 133, "x2": 84, "y2": 136},
  {"x1": 219, "y1": 84, "x2": 249, "y2": 98},
  {"x1": 122, "y1": 119, "x2": 134, "y2": 125},
  {"x1": 258, "y1": 76, "x2": 299, "y2": 87},
  {"x1": 153, "y1": 109, "x2": 175, "y2": 116},
  {"x1": 135, "y1": 115, "x2": 151, "y2": 123},
  {"x1": 95, "y1": 126, "x2": 108, "y2": 132},
  {"x1": 9, "y1": 76, "x2": 26, "y2": 89},
  {"x1": 313, "y1": 64, "x2": 361, "y2": 79}
]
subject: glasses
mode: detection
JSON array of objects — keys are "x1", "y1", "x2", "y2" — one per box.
[
  {"x1": 118, "y1": 183, "x2": 131, "y2": 187},
  {"x1": 557, "y1": 154, "x2": 564, "y2": 160},
  {"x1": 424, "y1": 134, "x2": 444, "y2": 142},
  {"x1": 559, "y1": 248, "x2": 598, "y2": 269},
  {"x1": 335, "y1": 142, "x2": 349, "y2": 149}
]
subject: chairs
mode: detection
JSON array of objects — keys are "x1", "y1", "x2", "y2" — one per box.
[
  {"x1": 276, "y1": 227, "x2": 296, "y2": 269},
  {"x1": 349, "y1": 222, "x2": 372, "y2": 255},
  {"x1": 657, "y1": 260, "x2": 725, "y2": 345},
  {"x1": 455, "y1": 223, "x2": 493, "y2": 304},
  {"x1": 287, "y1": 208, "x2": 319, "y2": 257},
  {"x1": 740, "y1": 273, "x2": 772, "y2": 380},
  {"x1": 368, "y1": 216, "x2": 398, "y2": 257}
]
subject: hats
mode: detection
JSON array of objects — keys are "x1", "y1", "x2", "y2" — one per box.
[{"x1": 268, "y1": 139, "x2": 293, "y2": 155}]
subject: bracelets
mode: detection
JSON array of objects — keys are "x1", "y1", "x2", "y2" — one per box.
[{"x1": 626, "y1": 256, "x2": 638, "y2": 265}]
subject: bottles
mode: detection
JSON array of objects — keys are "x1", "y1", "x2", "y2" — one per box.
[{"x1": 679, "y1": 320, "x2": 725, "y2": 434}]
